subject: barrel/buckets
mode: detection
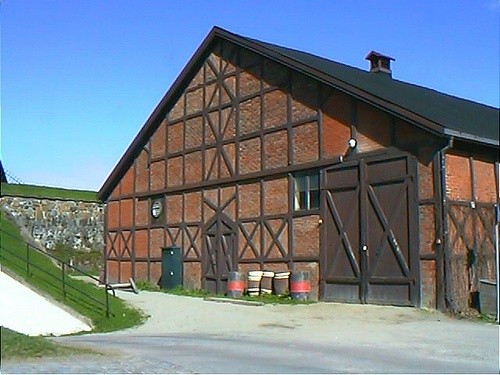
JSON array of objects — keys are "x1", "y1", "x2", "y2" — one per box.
[
  {"x1": 289, "y1": 270, "x2": 311, "y2": 301},
  {"x1": 228, "y1": 271, "x2": 246, "y2": 299}
]
